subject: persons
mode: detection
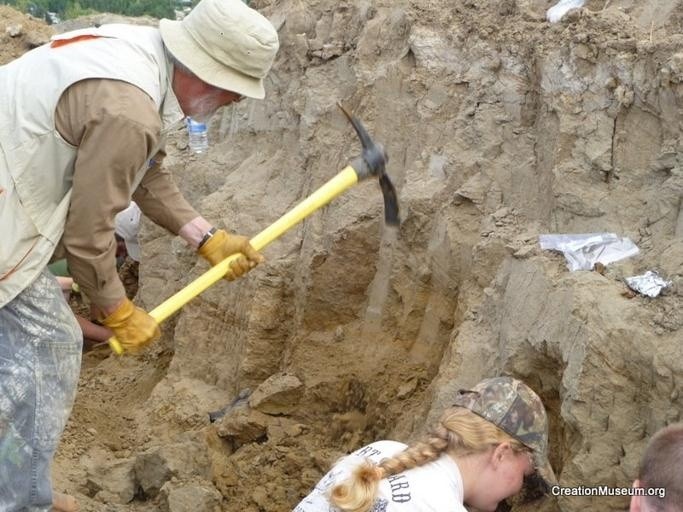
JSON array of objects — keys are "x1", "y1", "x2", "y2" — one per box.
[
  {"x1": 46, "y1": 200, "x2": 141, "y2": 341},
  {"x1": 0, "y1": 0, "x2": 281, "y2": 512},
  {"x1": 292, "y1": 376, "x2": 560, "y2": 512},
  {"x1": 629, "y1": 423, "x2": 683, "y2": 512}
]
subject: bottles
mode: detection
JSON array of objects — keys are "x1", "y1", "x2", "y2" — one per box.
[
  {"x1": 186, "y1": 114, "x2": 207, "y2": 154},
  {"x1": 546, "y1": 0, "x2": 584, "y2": 22}
]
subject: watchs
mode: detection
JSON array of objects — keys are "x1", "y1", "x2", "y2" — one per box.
[{"x1": 197, "y1": 227, "x2": 218, "y2": 249}]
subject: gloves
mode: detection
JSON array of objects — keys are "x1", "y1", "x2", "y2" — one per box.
[
  {"x1": 198, "y1": 229, "x2": 259, "y2": 281},
  {"x1": 98, "y1": 297, "x2": 160, "y2": 353}
]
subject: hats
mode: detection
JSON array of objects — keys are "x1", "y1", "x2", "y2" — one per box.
[
  {"x1": 451, "y1": 377, "x2": 558, "y2": 488},
  {"x1": 158, "y1": 0, "x2": 279, "y2": 100},
  {"x1": 112, "y1": 201, "x2": 142, "y2": 264}
]
